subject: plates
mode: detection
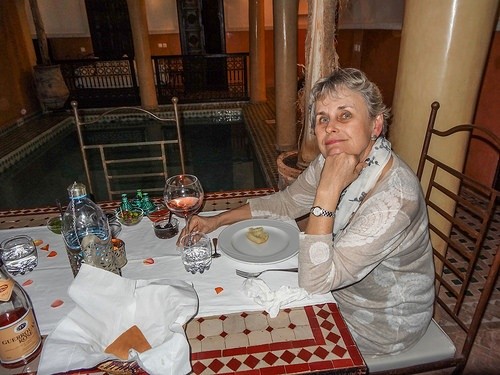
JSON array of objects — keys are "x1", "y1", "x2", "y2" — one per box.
[{"x1": 218, "y1": 218, "x2": 301, "y2": 265}]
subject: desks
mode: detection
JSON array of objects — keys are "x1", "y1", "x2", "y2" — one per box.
[{"x1": 0, "y1": 188, "x2": 369, "y2": 375}]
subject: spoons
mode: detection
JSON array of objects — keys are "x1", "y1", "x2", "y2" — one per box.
[
  {"x1": 210, "y1": 238, "x2": 221, "y2": 259},
  {"x1": 165, "y1": 211, "x2": 173, "y2": 228}
]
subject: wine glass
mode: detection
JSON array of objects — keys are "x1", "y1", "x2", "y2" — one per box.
[
  {"x1": 147, "y1": 199, "x2": 168, "y2": 227},
  {"x1": 164, "y1": 174, "x2": 205, "y2": 249}
]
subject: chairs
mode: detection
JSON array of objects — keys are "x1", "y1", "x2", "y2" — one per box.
[
  {"x1": 71, "y1": 97, "x2": 185, "y2": 201},
  {"x1": 360, "y1": 101, "x2": 500, "y2": 375}
]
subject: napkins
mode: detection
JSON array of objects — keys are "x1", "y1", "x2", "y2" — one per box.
[
  {"x1": 35, "y1": 262, "x2": 198, "y2": 375},
  {"x1": 242, "y1": 276, "x2": 308, "y2": 319}
]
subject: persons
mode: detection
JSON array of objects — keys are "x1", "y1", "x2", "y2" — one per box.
[{"x1": 176, "y1": 68, "x2": 436, "y2": 356}]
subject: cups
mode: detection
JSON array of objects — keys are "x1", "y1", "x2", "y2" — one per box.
[
  {"x1": 112, "y1": 239, "x2": 126, "y2": 268},
  {"x1": 1, "y1": 235, "x2": 38, "y2": 276},
  {"x1": 181, "y1": 233, "x2": 213, "y2": 274}
]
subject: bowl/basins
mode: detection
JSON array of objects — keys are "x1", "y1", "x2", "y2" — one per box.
[
  {"x1": 102, "y1": 210, "x2": 117, "y2": 223},
  {"x1": 47, "y1": 215, "x2": 64, "y2": 234},
  {"x1": 153, "y1": 217, "x2": 179, "y2": 240},
  {"x1": 108, "y1": 222, "x2": 122, "y2": 238},
  {"x1": 116, "y1": 208, "x2": 143, "y2": 226}
]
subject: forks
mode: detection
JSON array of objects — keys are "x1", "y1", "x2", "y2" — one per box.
[{"x1": 236, "y1": 268, "x2": 299, "y2": 279}]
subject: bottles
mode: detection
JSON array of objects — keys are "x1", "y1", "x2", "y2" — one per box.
[
  {"x1": 0, "y1": 260, "x2": 43, "y2": 368},
  {"x1": 120, "y1": 194, "x2": 130, "y2": 213},
  {"x1": 131, "y1": 190, "x2": 144, "y2": 213},
  {"x1": 141, "y1": 193, "x2": 152, "y2": 216},
  {"x1": 62, "y1": 183, "x2": 120, "y2": 280}
]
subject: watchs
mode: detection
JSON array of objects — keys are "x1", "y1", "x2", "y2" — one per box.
[{"x1": 310, "y1": 206, "x2": 335, "y2": 218}]
